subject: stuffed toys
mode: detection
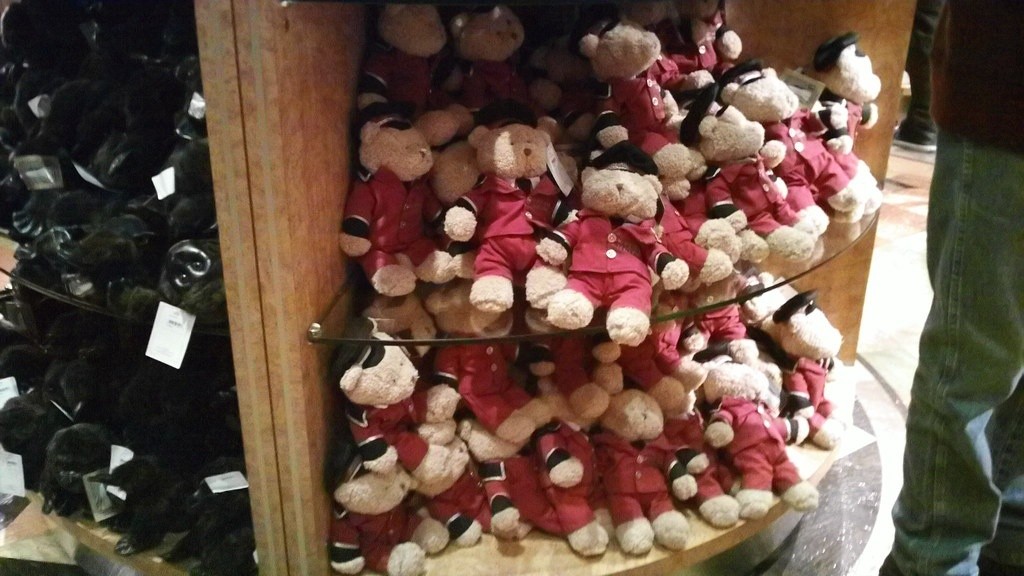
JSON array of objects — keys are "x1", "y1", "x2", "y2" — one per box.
[
  {"x1": 327, "y1": 1, "x2": 884, "y2": 576},
  {"x1": 0, "y1": 0, "x2": 260, "y2": 576}
]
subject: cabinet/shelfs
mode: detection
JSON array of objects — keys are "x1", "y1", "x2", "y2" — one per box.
[
  {"x1": 230, "y1": 0, "x2": 917, "y2": 576},
  {"x1": 0, "y1": 0, "x2": 289, "y2": 576}
]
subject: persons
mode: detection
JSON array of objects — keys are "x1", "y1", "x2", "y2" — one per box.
[{"x1": 879, "y1": 0, "x2": 1024, "y2": 576}]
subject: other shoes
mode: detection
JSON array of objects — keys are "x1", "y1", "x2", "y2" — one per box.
[{"x1": 892, "y1": 128, "x2": 937, "y2": 151}]
